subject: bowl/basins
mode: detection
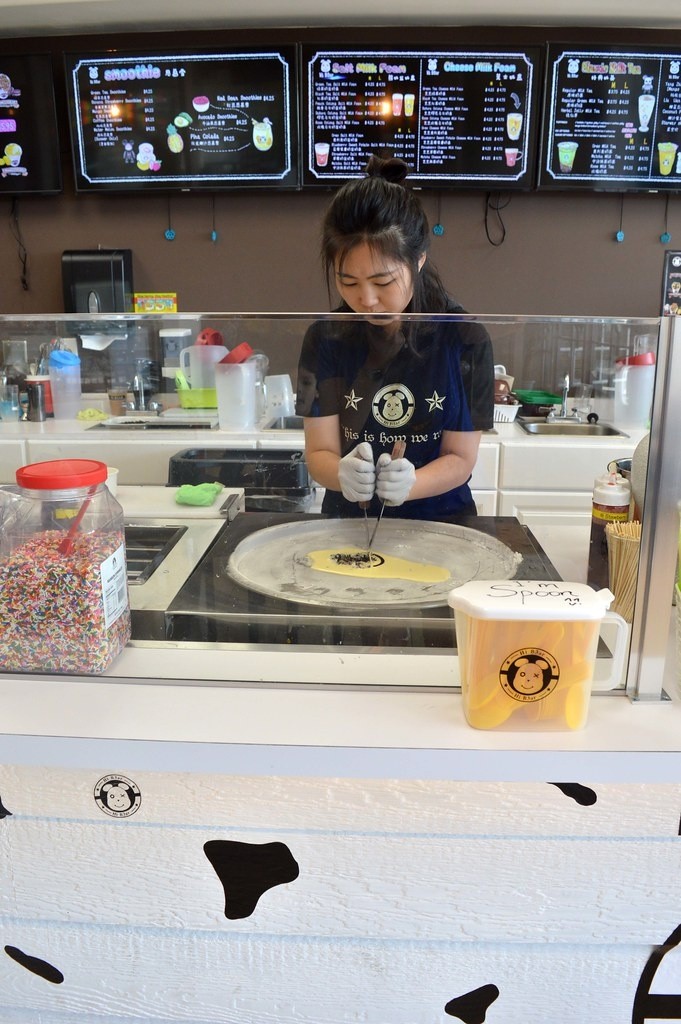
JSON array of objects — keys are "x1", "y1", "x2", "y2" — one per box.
[
  {"x1": 192, "y1": 97, "x2": 210, "y2": 113},
  {"x1": 493, "y1": 380, "x2": 516, "y2": 404}
]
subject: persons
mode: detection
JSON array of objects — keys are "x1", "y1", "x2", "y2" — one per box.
[{"x1": 293, "y1": 156, "x2": 497, "y2": 518}]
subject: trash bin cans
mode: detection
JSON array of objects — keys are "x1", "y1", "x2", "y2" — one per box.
[{"x1": 165, "y1": 448, "x2": 317, "y2": 513}]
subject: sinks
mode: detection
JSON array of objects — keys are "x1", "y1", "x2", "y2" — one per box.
[
  {"x1": 525, "y1": 423, "x2": 621, "y2": 436},
  {"x1": 85, "y1": 416, "x2": 218, "y2": 431}
]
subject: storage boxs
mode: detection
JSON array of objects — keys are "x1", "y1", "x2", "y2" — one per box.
[
  {"x1": 166, "y1": 448, "x2": 314, "y2": 496},
  {"x1": 494, "y1": 400, "x2": 521, "y2": 425},
  {"x1": 174, "y1": 387, "x2": 217, "y2": 408},
  {"x1": 453, "y1": 580, "x2": 612, "y2": 731}
]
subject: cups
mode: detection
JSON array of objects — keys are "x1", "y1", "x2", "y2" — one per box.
[
  {"x1": 636, "y1": 95, "x2": 655, "y2": 133},
  {"x1": 658, "y1": 143, "x2": 678, "y2": 175},
  {"x1": 263, "y1": 374, "x2": 296, "y2": 418},
  {"x1": 392, "y1": 93, "x2": 403, "y2": 116},
  {"x1": 404, "y1": 93, "x2": 415, "y2": 117},
  {"x1": 557, "y1": 141, "x2": 579, "y2": 173},
  {"x1": 25, "y1": 375, "x2": 53, "y2": 416},
  {"x1": 48, "y1": 350, "x2": 81, "y2": 419},
  {"x1": 0, "y1": 74, "x2": 11, "y2": 99},
  {"x1": 603, "y1": 528, "x2": 639, "y2": 624},
  {"x1": 507, "y1": 112, "x2": 523, "y2": 140},
  {"x1": 253, "y1": 123, "x2": 273, "y2": 150},
  {"x1": 5, "y1": 144, "x2": 22, "y2": 166},
  {"x1": 180, "y1": 343, "x2": 230, "y2": 389},
  {"x1": 107, "y1": 388, "x2": 128, "y2": 416},
  {"x1": 315, "y1": 142, "x2": 330, "y2": 166},
  {"x1": 505, "y1": 147, "x2": 523, "y2": 167},
  {"x1": 612, "y1": 364, "x2": 656, "y2": 430},
  {"x1": 215, "y1": 361, "x2": 257, "y2": 430},
  {"x1": 105, "y1": 467, "x2": 119, "y2": 501},
  {"x1": 0, "y1": 385, "x2": 19, "y2": 423}
]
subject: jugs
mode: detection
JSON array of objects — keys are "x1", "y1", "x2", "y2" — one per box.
[{"x1": 445, "y1": 578, "x2": 630, "y2": 732}]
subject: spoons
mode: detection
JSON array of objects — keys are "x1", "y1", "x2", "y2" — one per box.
[
  {"x1": 463, "y1": 612, "x2": 595, "y2": 730},
  {"x1": 56, "y1": 484, "x2": 97, "y2": 557}
]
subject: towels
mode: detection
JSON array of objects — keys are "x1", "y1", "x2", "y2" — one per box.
[{"x1": 175, "y1": 480, "x2": 224, "y2": 507}]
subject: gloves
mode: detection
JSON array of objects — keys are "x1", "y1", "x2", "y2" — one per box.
[
  {"x1": 338, "y1": 442, "x2": 376, "y2": 502},
  {"x1": 376, "y1": 453, "x2": 416, "y2": 506}
]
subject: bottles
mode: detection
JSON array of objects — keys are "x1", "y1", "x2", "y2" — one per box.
[
  {"x1": 587, "y1": 469, "x2": 631, "y2": 592},
  {"x1": 0, "y1": 458, "x2": 133, "y2": 674}
]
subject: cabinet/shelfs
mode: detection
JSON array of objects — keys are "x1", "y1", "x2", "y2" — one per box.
[{"x1": 472, "y1": 492, "x2": 596, "y2": 582}]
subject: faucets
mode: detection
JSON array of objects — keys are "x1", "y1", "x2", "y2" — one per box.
[
  {"x1": 560, "y1": 371, "x2": 570, "y2": 418},
  {"x1": 134, "y1": 372, "x2": 146, "y2": 410}
]
